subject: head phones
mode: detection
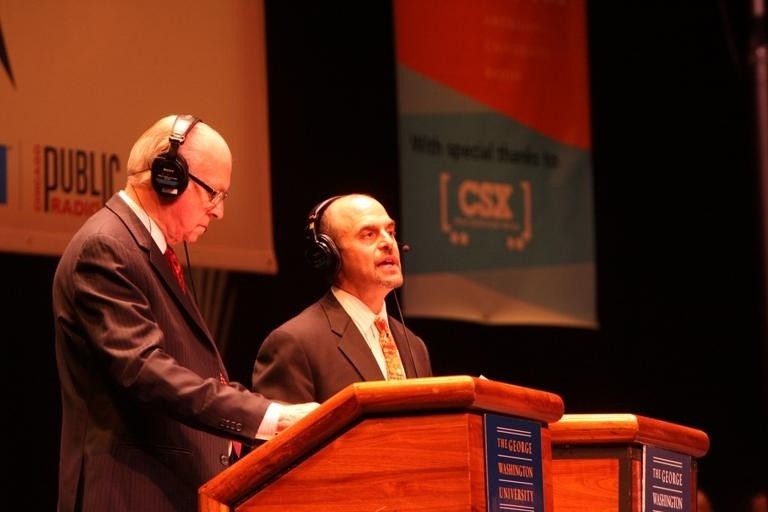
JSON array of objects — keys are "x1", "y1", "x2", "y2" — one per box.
[
  {"x1": 150, "y1": 113, "x2": 202, "y2": 199},
  {"x1": 304, "y1": 195, "x2": 342, "y2": 276}
]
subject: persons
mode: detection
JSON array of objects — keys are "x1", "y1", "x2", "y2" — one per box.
[
  {"x1": 251, "y1": 189, "x2": 433, "y2": 459},
  {"x1": 52, "y1": 115, "x2": 322, "y2": 511}
]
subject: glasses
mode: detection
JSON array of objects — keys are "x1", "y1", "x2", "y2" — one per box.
[{"x1": 188, "y1": 173, "x2": 231, "y2": 205}]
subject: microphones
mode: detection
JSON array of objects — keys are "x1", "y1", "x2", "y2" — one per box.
[{"x1": 402, "y1": 244, "x2": 411, "y2": 253}]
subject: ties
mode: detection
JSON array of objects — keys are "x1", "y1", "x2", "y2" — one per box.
[
  {"x1": 164, "y1": 243, "x2": 243, "y2": 459},
  {"x1": 374, "y1": 315, "x2": 404, "y2": 381}
]
